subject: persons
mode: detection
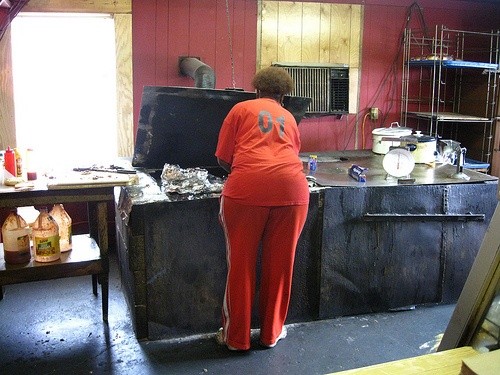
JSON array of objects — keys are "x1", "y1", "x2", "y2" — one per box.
[{"x1": 215, "y1": 64, "x2": 310, "y2": 351}]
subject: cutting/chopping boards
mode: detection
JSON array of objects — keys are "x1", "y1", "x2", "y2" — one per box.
[{"x1": 46, "y1": 172, "x2": 130, "y2": 190}]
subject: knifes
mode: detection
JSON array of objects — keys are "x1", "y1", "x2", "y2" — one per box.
[{"x1": 91, "y1": 168, "x2": 137, "y2": 174}]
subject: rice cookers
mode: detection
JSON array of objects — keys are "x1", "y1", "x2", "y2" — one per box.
[
  {"x1": 372, "y1": 121, "x2": 412, "y2": 154},
  {"x1": 399, "y1": 131, "x2": 437, "y2": 165}
]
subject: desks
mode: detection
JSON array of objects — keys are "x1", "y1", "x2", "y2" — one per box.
[{"x1": 0, "y1": 179, "x2": 115, "y2": 323}]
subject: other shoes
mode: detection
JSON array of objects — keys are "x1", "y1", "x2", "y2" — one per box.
[
  {"x1": 267, "y1": 325, "x2": 287, "y2": 348},
  {"x1": 215, "y1": 328, "x2": 239, "y2": 350}
]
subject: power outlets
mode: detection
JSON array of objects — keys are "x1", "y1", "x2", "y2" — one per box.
[{"x1": 371, "y1": 107, "x2": 378, "y2": 120}]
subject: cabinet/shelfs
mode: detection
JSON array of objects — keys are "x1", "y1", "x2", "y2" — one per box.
[{"x1": 400, "y1": 23, "x2": 500, "y2": 174}]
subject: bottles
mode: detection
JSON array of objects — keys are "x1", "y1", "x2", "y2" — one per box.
[
  {"x1": 5, "y1": 146, "x2": 16, "y2": 177},
  {"x1": 26, "y1": 148, "x2": 37, "y2": 181},
  {"x1": 14, "y1": 148, "x2": 22, "y2": 177},
  {"x1": 2, "y1": 207, "x2": 31, "y2": 264},
  {"x1": 31, "y1": 206, "x2": 60, "y2": 263},
  {"x1": 49, "y1": 203, "x2": 72, "y2": 253}
]
